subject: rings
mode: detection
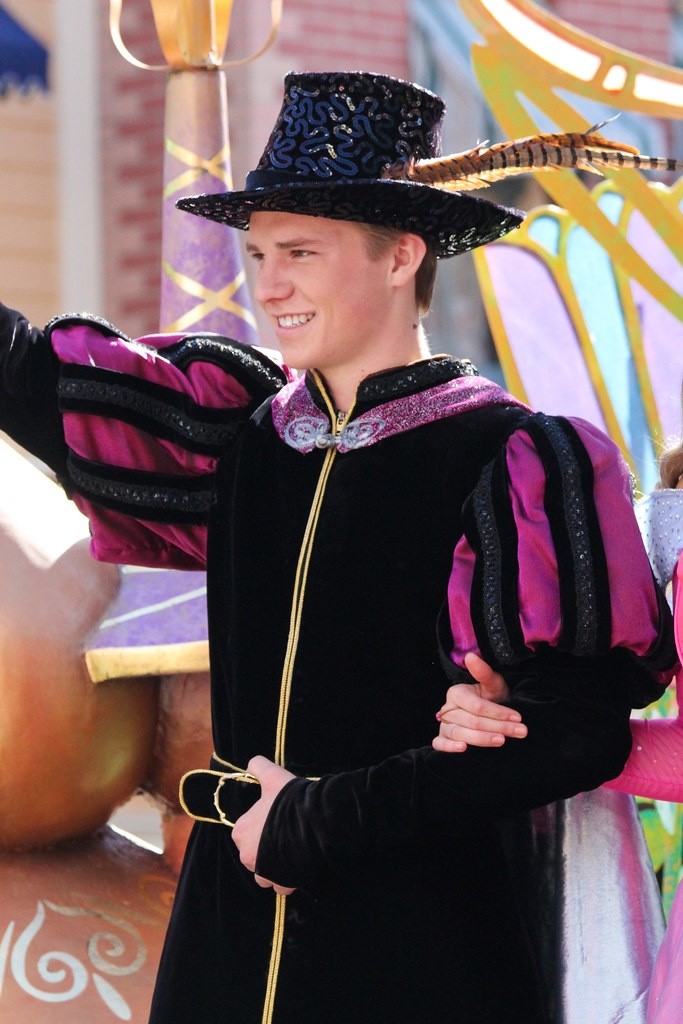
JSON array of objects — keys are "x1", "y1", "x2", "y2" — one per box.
[{"x1": 436, "y1": 703, "x2": 458, "y2": 725}]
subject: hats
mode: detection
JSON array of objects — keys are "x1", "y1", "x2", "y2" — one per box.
[{"x1": 173, "y1": 71, "x2": 683, "y2": 258}]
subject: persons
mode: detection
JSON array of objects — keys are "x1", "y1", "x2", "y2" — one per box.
[
  {"x1": 432, "y1": 444, "x2": 681, "y2": 1024},
  {"x1": 1, "y1": 70, "x2": 681, "y2": 1024}
]
